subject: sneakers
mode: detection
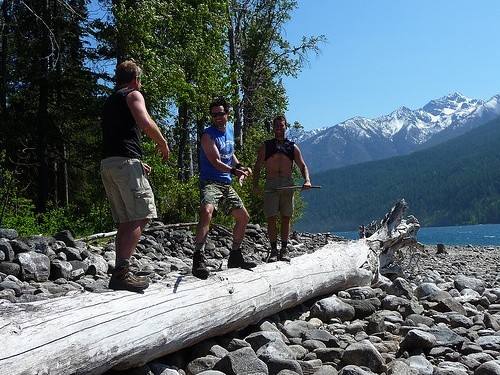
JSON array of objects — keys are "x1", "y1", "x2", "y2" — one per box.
[
  {"x1": 108, "y1": 266, "x2": 149, "y2": 291},
  {"x1": 192, "y1": 252, "x2": 210, "y2": 275},
  {"x1": 227, "y1": 248, "x2": 257, "y2": 269},
  {"x1": 268, "y1": 250, "x2": 278, "y2": 262},
  {"x1": 280, "y1": 249, "x2": 290, "y2": 262}
]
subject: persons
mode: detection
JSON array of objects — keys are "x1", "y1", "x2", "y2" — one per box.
[
  {"x1": 191, "y1": 99, "x2": 256, "y2": 278},
  {"x1": 100, "y1": 61, "x2": 169, "y2": 290},
  {"x1": 253, "y1": 116, "x2": 311, "y2": 263}
]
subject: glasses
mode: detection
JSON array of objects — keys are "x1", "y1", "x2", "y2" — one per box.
[{"x1": 210, "y1": 112, "x2": 226, "y2": 118}]
